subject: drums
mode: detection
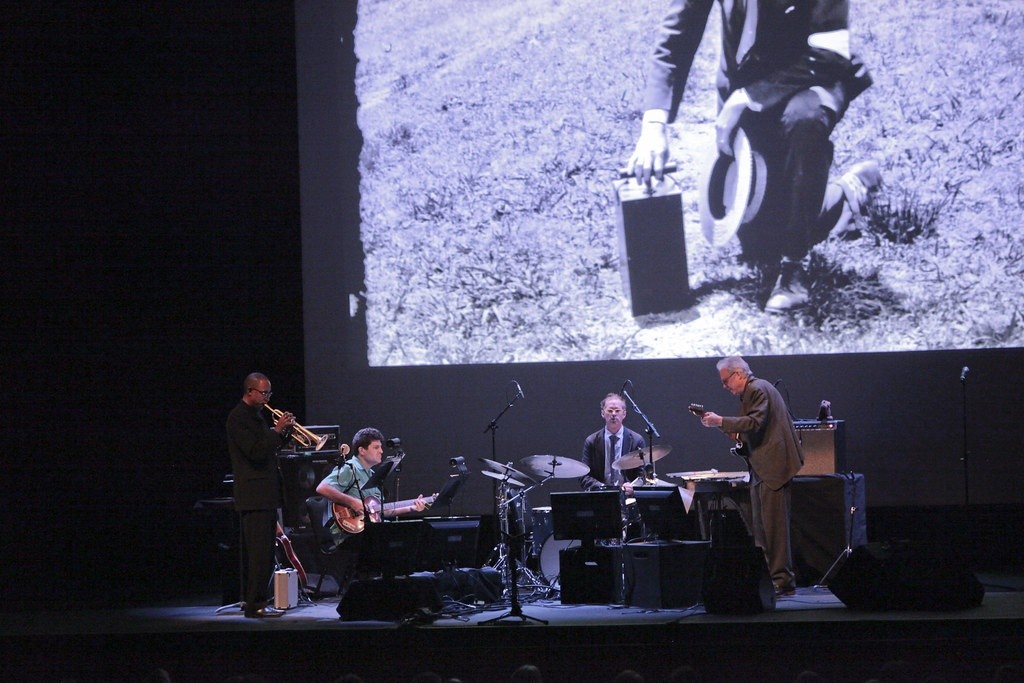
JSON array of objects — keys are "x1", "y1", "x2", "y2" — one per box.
[
  {"x1": 539, "y1": 531, "x2": 581, "y2": 591},
  {"x1": 624, "y1": 497, "x2": 646, "y2": 543},
  {"x1": 532, "y1": 506, "x2": 554, "y2": 558}
]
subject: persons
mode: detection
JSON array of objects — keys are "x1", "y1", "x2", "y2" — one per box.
[
  {"x1": 227, "y1": 372, "x2": 296, "y2": 618},
  {"x1": 316, "y1": 428, "x2": 427, "y2": 565},
  {"x1": 579, "y1": 394, "x2": 648, "y2": 522},
  {"x1": 700, "y1": 357, "x2": 804, "y2": 597},
  {"x1": 627, "y1": 0, "x2": 882, "y2": 317}
]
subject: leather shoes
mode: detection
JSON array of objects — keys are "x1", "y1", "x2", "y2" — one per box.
[
  {"x1": 245, "y1": 607, "x2": 282, "y2": 618},
  {"x1": 265, "y1": 606, "x2": 285, "y2": 614}
]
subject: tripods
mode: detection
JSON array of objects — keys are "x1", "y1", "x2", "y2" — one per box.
[{"x1": 477, "y1": 390, "x2": 549, "y2": 625}]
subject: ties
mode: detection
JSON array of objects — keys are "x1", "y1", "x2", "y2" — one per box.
[{"x1": 610, "y1": 435, "x2": 620, "y2": 484}]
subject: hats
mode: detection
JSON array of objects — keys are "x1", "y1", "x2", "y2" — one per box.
[{"x1": 699, "y1": 128, "x2": 767, "y2": 246}]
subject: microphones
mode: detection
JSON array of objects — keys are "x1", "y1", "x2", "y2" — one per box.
[
  {"x1": 960, "y1": 366, "x2": 970, "y2": 382},
  {"x1": 619, "y1": 381, "x2": 628, "y2": 396},
  {"x1": 337, "y1": 443, "x2": 350, "y2": 479},
  {"x1": 513, "y1": 381, "x2": 524, "y2": 399},
  {"x1": 773, "y1": 380, "x2": 780, "y2": 387}
]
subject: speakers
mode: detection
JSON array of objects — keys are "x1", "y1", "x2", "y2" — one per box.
[
  {"x1": 792, "y1": 419, "x2": 846, "y2": 476},
  {"x1": 828, "y1": 542, "x2": 985, "y2": 611},
  {"x1": 275, "y1": 449, "x2": 341, "y2": 527}
]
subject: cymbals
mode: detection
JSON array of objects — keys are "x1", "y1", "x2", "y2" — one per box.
[
  {"x1": 520, "y1": 455, "x2": 591, "y2": 479},
  {"x1": 611, "y1": 445, "x2": 673, "y2": 470},
  {"x1": 478, "y1": 457, "x2": 539, "y2": 486},
  {"x1": 481, "y1": 471, "x2": 525, "y2": 486}
]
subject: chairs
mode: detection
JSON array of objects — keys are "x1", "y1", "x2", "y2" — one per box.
[{"x1": 305, "y1": 496, "x2": 360, "y2": 599}]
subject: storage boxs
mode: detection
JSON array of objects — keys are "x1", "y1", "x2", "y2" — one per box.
[{"x1": 275, "y1": 568, "x2": 299, "y2": 609}]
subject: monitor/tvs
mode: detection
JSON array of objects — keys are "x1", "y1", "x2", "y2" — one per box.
[
  {"x1": 632, "y1": 485, "x2": 691, "y2": 544},
  {"x1": 364, "y1": 515, "x2": 481, "y2": 580},
  {"x1": 550, "y1": 490, "x2": 623, "y2": 548}
]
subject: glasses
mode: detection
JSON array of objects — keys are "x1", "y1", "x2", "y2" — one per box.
[
  {"x1": 253, "y1": 388, "x2": 273, "y2": 397},
  {"x1": 722, "y1": 372, "x2": 735, "y2": 385}
]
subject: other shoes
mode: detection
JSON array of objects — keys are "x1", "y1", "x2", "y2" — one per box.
[{"x1": 776, "y1": 579, "x2": 796, "y2": 595}]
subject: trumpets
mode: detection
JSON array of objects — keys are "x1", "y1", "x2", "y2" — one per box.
[{"x1": 262, "y1": 402, "x2": 328, "y2": 452}]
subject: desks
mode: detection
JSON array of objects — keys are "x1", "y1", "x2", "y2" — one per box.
[{"x1": 676, "y1": 472, "x2": 867, "y2": 587}]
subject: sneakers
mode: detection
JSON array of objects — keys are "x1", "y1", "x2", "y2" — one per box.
[
  {"x1": 763, "y1": 260, "x2": 811, "y2": 315},
  {"x1": 851, "y1": 159, "x2": 908, "y2": 238}
]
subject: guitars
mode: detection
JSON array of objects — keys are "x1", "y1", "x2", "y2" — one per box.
[
  {"x1": 275, "y1": 521, "x2": 308, "y2": 589},
  {"x1": 332, "y1": 492, "x2": 440, "y2": 536},
  {"x1": 688, "y1": 402, "x2": 751, "y2": 470}
]
